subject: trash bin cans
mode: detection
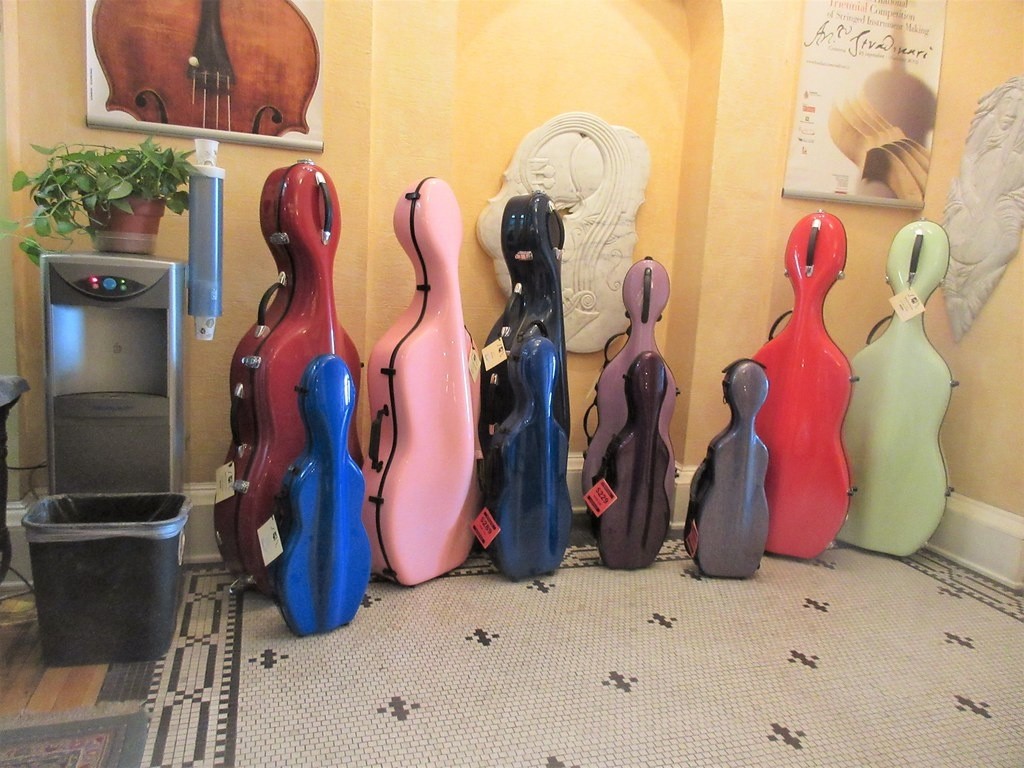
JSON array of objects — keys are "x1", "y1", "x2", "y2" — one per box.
[{"x1": 22, "y1": 492, "x2": 192, "y2": 667}]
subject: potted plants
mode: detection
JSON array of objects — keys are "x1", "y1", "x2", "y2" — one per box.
[{"x1": 0, "y1": 130, "x2": 209, "y2": 266}]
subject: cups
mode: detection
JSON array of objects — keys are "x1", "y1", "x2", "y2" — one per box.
[
  {"x1": 194, "y1": 316, "x2": 216, "y2": 341},
  {"x1": 194, "y1": 138, "x2": 219, "y2": 166}
]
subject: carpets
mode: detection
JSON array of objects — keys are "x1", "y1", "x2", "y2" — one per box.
[{"x1": 0, "y1": 699, "x2": 149, "y2": 768}]
